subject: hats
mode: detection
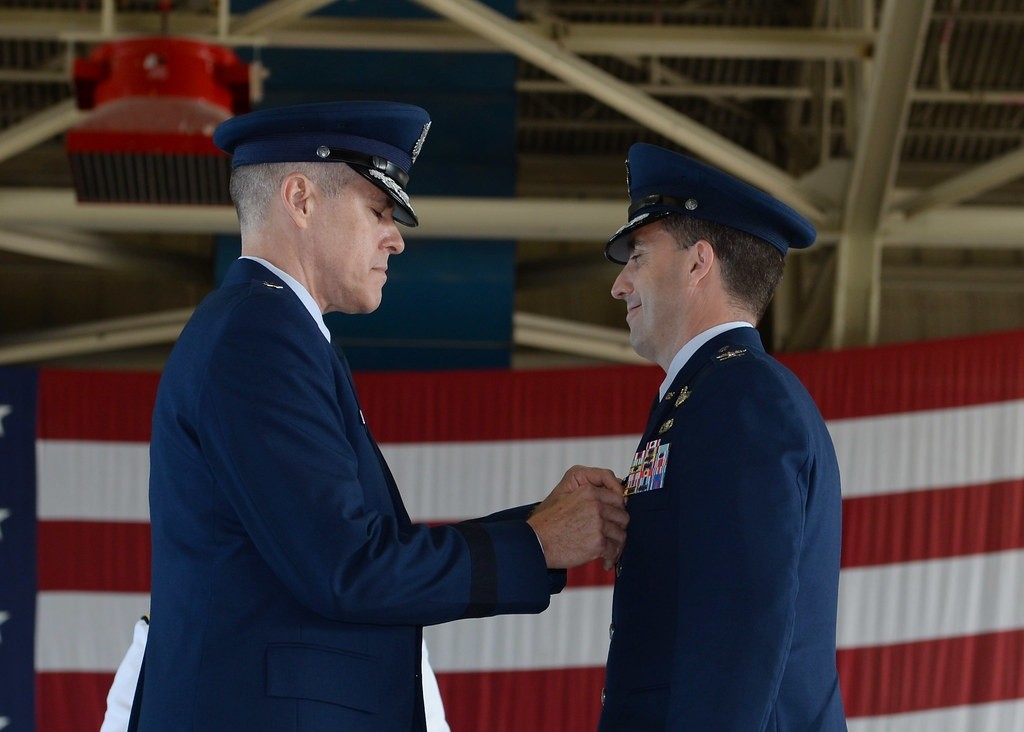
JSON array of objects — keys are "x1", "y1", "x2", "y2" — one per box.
[
  {"x1": 605, "y1": 142, "x2": 817, "y2": 265},
  {"x1": 214, "y1": 100, "x2": 432, "y2": 227}
]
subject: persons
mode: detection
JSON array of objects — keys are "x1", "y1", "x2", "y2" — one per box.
[
  {"x1": 598, "y1": 143, "x2": 847, "y2": 730},
  {"x1": 126, "y1": 99, "x2": 628, "y2": 732}
]
